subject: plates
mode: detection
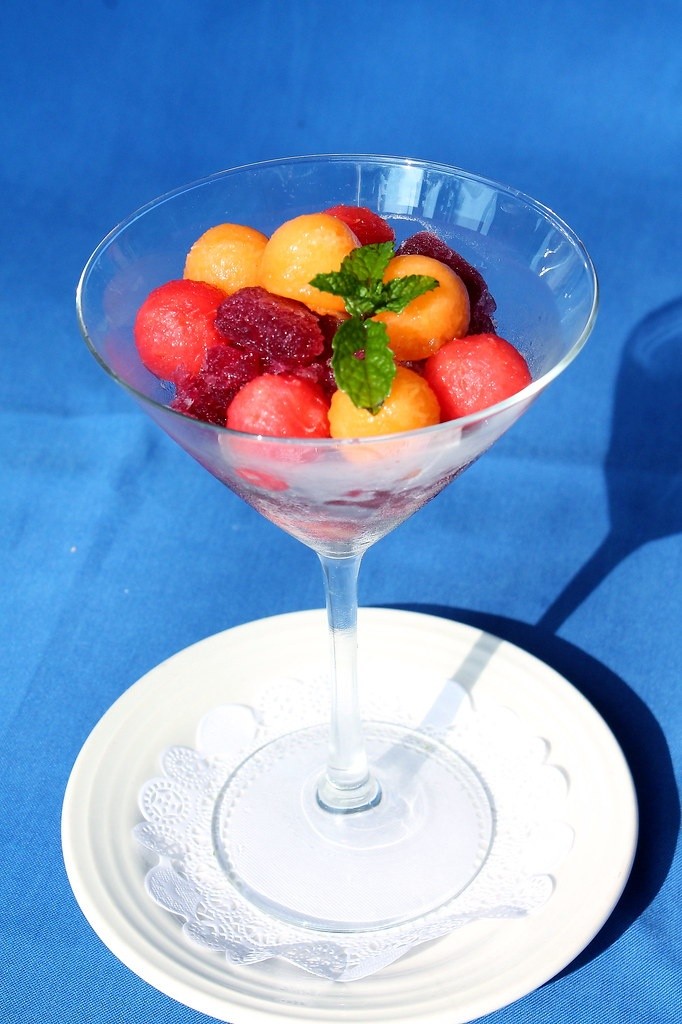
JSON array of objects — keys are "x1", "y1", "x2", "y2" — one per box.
[{"x1": 61, "y1": 609, "x2": 639, "y2": 1024}]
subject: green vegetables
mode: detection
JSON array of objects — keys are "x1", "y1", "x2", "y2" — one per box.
[{"x1": 313, "y1": 236, "x2": 439, "y2": 413}]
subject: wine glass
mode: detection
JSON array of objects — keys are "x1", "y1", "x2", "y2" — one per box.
[{"x1": 76, "y1": 153, "x2": 600, "y2": 935}]
